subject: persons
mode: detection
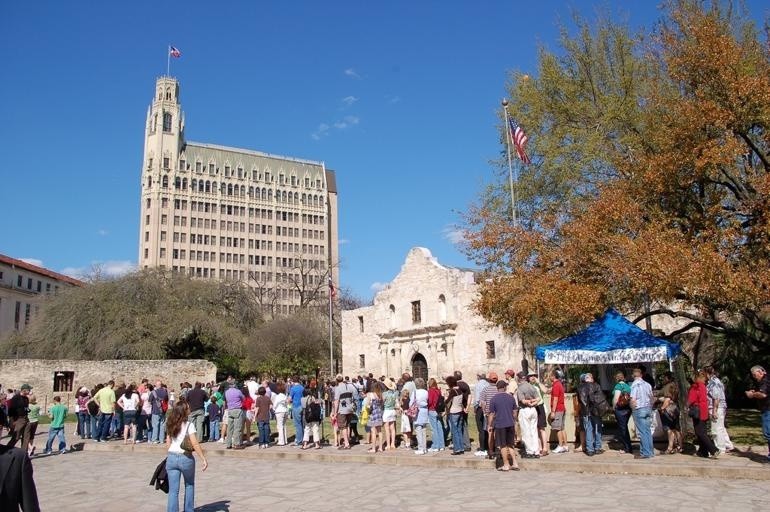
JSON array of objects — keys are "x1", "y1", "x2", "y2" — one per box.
[
  {"x1": 324, "y1": 371, "x2": 472, "y2": 456},
  {"x1": 0, "y1": 383, "x2": 20, "y2": 437},
  {"x1": 43, "y1": 396, "x2": 68, "y2": 455},
  {"x1": 0, "y1": 408, "x2": 41, "y2": 512},
  {"x1": 75, "y1": 378, "x2": 169, "y2": 444},
  {"x1": 6, "y1": 384, "x2": 34, "y2": 452},
  {"x1": 165, "y1": 401, "x2": 208, "y2": 512},
  {"x1": 744, "y1": 364, "x2": 770, "y2": 460},
  {"x1": 27, "y1": 394, "x2": 40, "y2": 456},
  {"x1": 473, "y1": 368, "x2": 567, "y2": 470},
  {"x1": 178, "y1": 373, "x2": 326, "y2": 450},
  {"x1": 577, "y1": 367, "x2": 734, "y2": 458}
]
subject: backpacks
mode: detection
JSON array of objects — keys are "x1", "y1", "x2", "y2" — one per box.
[
  {"x1": 405, "y1": 390, "x2": 420, "y2": 419},
  {"x1": 587, "y1": 387, "x2": 608, "y2": 419}
]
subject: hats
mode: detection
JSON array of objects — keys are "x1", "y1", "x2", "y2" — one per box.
[
  {"x1": 478, "y1": 366, "x2": 539, "y2": 387},
  {"x1": 382, "y1": 377, "x2": 396, "y2": 390},
  {"x1": 19, "y1": 383, "x2": 35, "y2": 390}
]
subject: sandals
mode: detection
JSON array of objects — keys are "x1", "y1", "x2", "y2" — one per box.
[{"x1": 661, "y1": 447, "x2": 686, "y2": 454}]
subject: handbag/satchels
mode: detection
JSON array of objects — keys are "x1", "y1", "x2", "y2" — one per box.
[
  {"x1": 360, "y1": 409, "x2": 369, "y2": 428},
  {"x1": 180, "y1": 437, "x2": 195, "y2": 454},
  {"x1": 305, "y1": 402, "x2": 322, "y2": 423},
  {"x1": 155, "y1": 396, "x2": 168, "y2": 415}
]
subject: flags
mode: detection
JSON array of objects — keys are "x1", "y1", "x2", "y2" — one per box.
[
  {"x1": 508, "y1": 111, "x2": 531, "y2": 164},
  {"x1": 170, "y1": 46, "x2": 183, "y2": 59},
  {"x1": 328, "y1": 272, "x2": 335, "y2": 298}
]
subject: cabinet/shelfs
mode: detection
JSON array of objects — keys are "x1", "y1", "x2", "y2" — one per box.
[{"x1": 543, "y1": 393, "x2": 579, "y2": 443}]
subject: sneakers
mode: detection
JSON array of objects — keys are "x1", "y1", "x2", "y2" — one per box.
[
  {"x1": 259, "y1": 441, "x2": 289, "y2": 449},
  {"x1": 585, "y1": 449, "x2": 605, "y2": 455},
  {"x1": 290, "y1": 440, "x2": 320, "y2": 449},
  {"x1": 29, "y1": 445, "x2": 35, "y2": 456},
  {"x1": 60, "y1": 449, "x2": 68, "y2": 455},
  {"x1": 473, "y1": 449, "x2": 489, "y2": 457},
  {"x1": 44, "y1": 449, "x2": 53, "y2": 453},
  {"x1": 496, "y1": 465, "x2": 521, "y2": 472},
  {"x1": 551, "y1": 443, "x2": 569, "y2": 454},
  {"x1": 73, "y1": 430, "x2": 166, "y2": 445},
  {"x1": 364, "y1": 441, "x2": 470, "y2": 455},
  {"x1": 331, "y1": 439, "x2": 359, "y2": 450},
  {"x1": 633, "y1": 453, "x2": 653, "y2": 459},
  {"x1": 199, "y1": 439, "x2": 253, "y2": 449},
  {"x1": 526, "y1": 451, "x2": 549, "y2": 459},
  {"x1": 692, "y1": 441, "x2": 734, "y2": 458}
]
